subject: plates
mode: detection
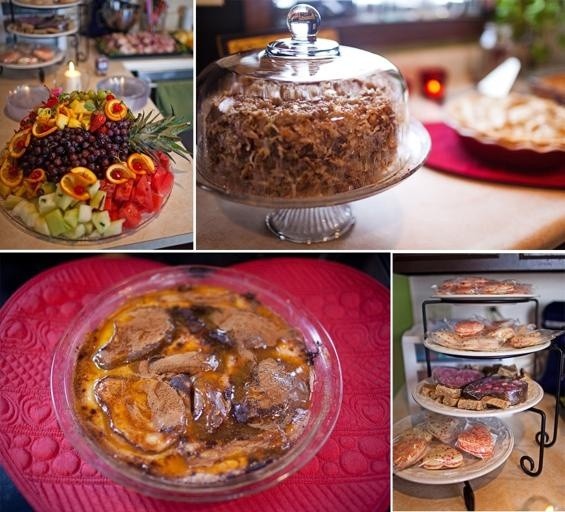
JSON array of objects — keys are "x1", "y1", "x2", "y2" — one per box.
[
  {"x1": 1, "y1": 1, "x2": 80, "y2": 72},
  {"x1": 393, "y1": 276, "x2": 553, "y2": 486},
  {"x1": 94, "y1": 27, "x2": 194, "y2": 61},
  {"x1": 436, "y1": 87, "x2": 564, "y2": 170},
  {"x1": 1, "y1": 160, "x2": 175, "y2": 245},
  {"x1": 49, "y1": 263, "x2": 347, "y2": 503}
]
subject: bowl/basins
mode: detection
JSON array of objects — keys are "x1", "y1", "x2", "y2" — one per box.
[{"x1": 92, "y1": 2, "x2": 148, "y2": 33}]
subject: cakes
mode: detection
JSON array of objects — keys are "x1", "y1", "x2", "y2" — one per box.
[
  {"x1": 435, "y1": 278, "x2": 520, "y2": 294},
  {"x1": 203, "y1": 76, "x2": 399, "y2": 199}
]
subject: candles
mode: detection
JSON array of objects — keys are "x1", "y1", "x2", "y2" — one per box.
[{"x1": 64, "y1": 61, "x2": 81, "y2": 91}]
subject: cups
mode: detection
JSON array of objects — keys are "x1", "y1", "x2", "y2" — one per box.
[
  {"x1": 4, "y1": 83, "x2": 50, "y2": 122},
  {"x1": 96, "y1": 76, "x2": 149, "y2": 111}
]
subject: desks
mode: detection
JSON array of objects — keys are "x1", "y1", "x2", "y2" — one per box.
[
  {"x1": 0, "y1": 41, "x2": 192, "y2": 249},
  {"x1": 197, "y1": 168, "x2": 565, "y2": 249}
]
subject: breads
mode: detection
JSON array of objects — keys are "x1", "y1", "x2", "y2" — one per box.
[{"x1": 419, "y1": 364, "x2": 528, "y2": 410}]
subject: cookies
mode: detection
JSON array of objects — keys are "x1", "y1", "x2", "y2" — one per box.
[
  {"x1": 393, "y1": 414, "x2": 495, "y2": 470},
  {"x1": 431, "y1": 318, "x2": 541, "y2": 351}
]
